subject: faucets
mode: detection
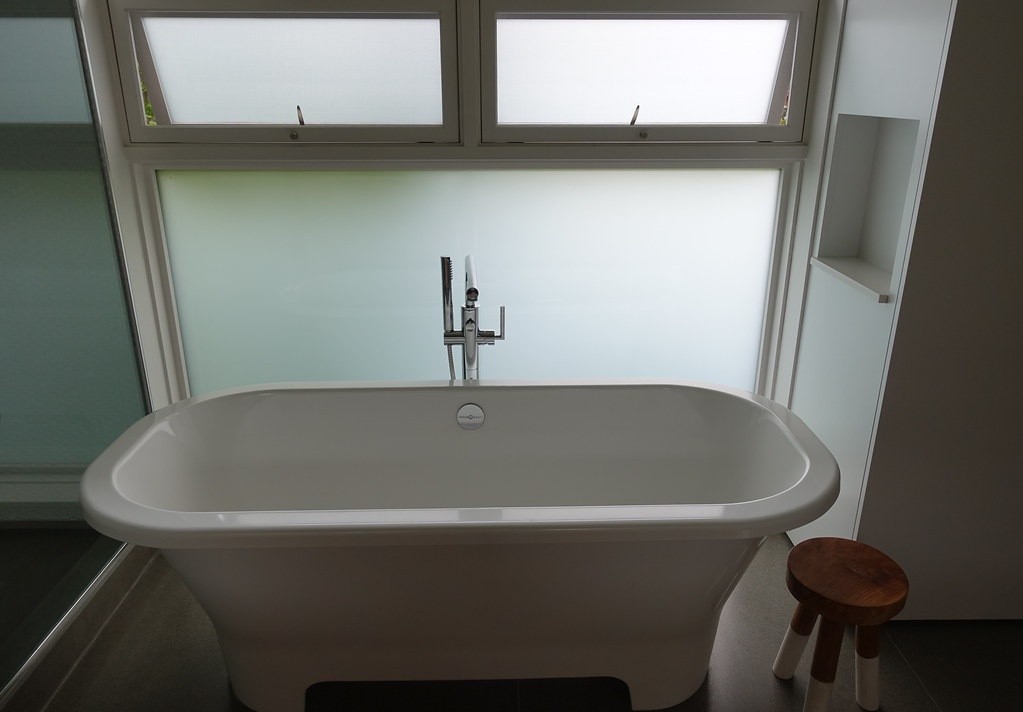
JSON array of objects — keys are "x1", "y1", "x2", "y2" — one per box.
[{"x1": 439, "y1": 252, "x2": 509, "y2": 381}]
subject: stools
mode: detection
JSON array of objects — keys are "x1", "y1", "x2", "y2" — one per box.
[{"x1": 772, "y1": 537, "x2": 910, "y2": 712}]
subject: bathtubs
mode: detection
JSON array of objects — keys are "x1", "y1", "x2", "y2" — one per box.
[{"x1": 77, "y1": 377, "x2": 843, "y2": 712}]
